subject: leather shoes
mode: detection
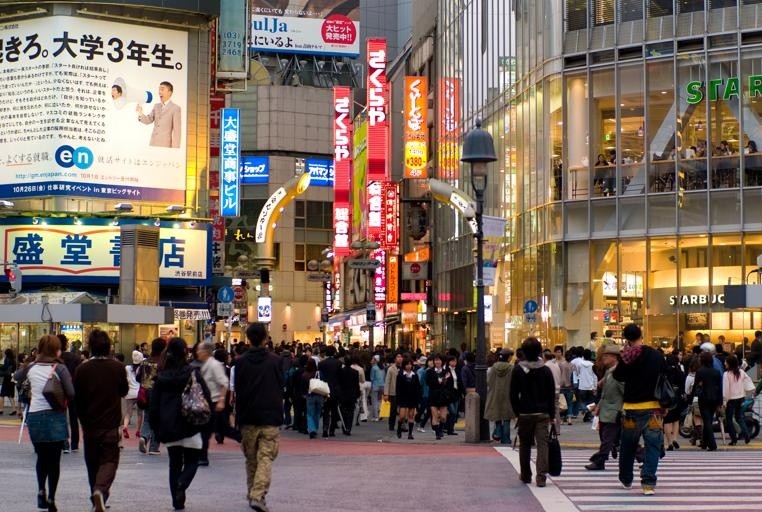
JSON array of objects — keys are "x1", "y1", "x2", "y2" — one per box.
[
  {"x1": 673, "y1": 440, "x2": 680, "y2": 449},
  {"x1": 666, "y1": 444, "x2": 673, "y2": 451},
  {"x1": 585, "y1": 463, "x2": 605, "y2": 470}
]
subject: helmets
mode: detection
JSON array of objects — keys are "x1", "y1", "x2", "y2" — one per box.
[{"x1": 700, "y1": 342, "x2": 716, "y2": 354}]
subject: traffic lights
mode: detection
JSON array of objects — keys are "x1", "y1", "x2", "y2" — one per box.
[{"x1": 6, "y1": 266, "x2": 21, "y2": 293}]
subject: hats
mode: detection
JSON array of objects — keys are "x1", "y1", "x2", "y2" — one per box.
[
  {"x1": 417, "y1": 356, "x2": 427, "y2": 365},
  {"x1": 131, "y1": 350, "x2": 147, "y2": 364},
  {"x1": 603, "y1": 344, "x2": 620, "y2": 355}
]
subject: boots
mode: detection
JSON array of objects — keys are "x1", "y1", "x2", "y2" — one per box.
[{"x1": 394, "y1": 420, "x2": 459, "y2": 439}]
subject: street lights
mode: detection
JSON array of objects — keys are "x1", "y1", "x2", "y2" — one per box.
[
  {"x1": 349, "y1": 242, "x2": 383, "y2": 305},
  {"x1": 305, "y1": 260, "x2": 330, "y2": 344},
  {"x1": 460, "y1": 117, "x2": 499, "y2": 443}
]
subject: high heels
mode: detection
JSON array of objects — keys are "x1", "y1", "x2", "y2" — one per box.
[
  {"x1": 745, "y1": 434, "x2": 750, "y2": 444},
  {"x1": 728, "y1": 439, "x2": 738, "y2": 445}
]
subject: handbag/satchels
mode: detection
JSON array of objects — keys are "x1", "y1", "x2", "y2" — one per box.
[
  {"x1": 379, "y1": 395, "x2": 391, "y2": 417},
  {"x1": 743, "y1": 376, "x2": 756, "y2": 397},
  {"x1": 655, "y1": 374, "x2": 677, "y2": 407},
  {"x1": 135, "y1": 386, "x2": 146, "y2": 408},
  {"x1": 309, "y1": 371, "x2": 330, "y2": 397},
  {"x1": 182, "y1": 373, "x2": 210, "y2": 425},
  {"x1": 42, "y1": 372, "x2": 65, "y2": 413},
  {"x1": 558, "y1": 393, "x2": 568, "y2": 412},
  {"x1": 572, "y1": 368, "x2": 578, "y2": 388},
  {"x1": 679, "y1": 393, "x2": 687, "y2": 409},
  {"x1": 549, "y1": 423, "x2": 561, "y2": 476}
]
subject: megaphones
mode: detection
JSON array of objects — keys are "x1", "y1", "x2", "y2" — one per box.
[{"x1": 112, "y1": 77, "x2": 154, "y2": 115}]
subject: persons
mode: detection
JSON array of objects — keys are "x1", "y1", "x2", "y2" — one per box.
[
  {"x1": 509, "y1": 335, "x2": 556, "y2": 487},
  {"x1": 135, "y1": 80, "x2": 182, "y2": 149},
  {"x1": 2, "y1": 328, "x2": 762, "y2": 471},
  {"x1": 611, "y1": 323, "x2": 668, "y2": 495},
  {"x1": 146, "y1": 336, "x2": 214, "y2": 511},
  {"x1": 13, "y1": 332, "x2": 77, "y2": 511},
  {"x1": 233, "y1": 321, "x2": 287, "y2": 511},
  {"x1": 69, "y1": 328, "x2": 130, "y2": 512},
  {"x1": 556, "y1": 134, "x2": 761, "y2": 195},
  {"x1": 111, "y1": 85, "x2": 122, "y2": 102}
]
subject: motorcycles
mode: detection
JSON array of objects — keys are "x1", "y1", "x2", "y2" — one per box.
[{"x1": 678, "y1": 375, "x2": 761, "y2": 441}]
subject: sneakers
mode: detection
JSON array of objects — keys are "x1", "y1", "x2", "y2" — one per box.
[
  {"x1": 620, "y1": 482, "x2": 632, "y2": 489},
  {"x1": 125, "y1": 426, "x2": 158, "y2": 454},
  {"x1": 642, "y1": 486, "x2": 654, "y2": 497},
  {"x1": 38, "y1": 490, "x2": 273, "y2": 510}
]
